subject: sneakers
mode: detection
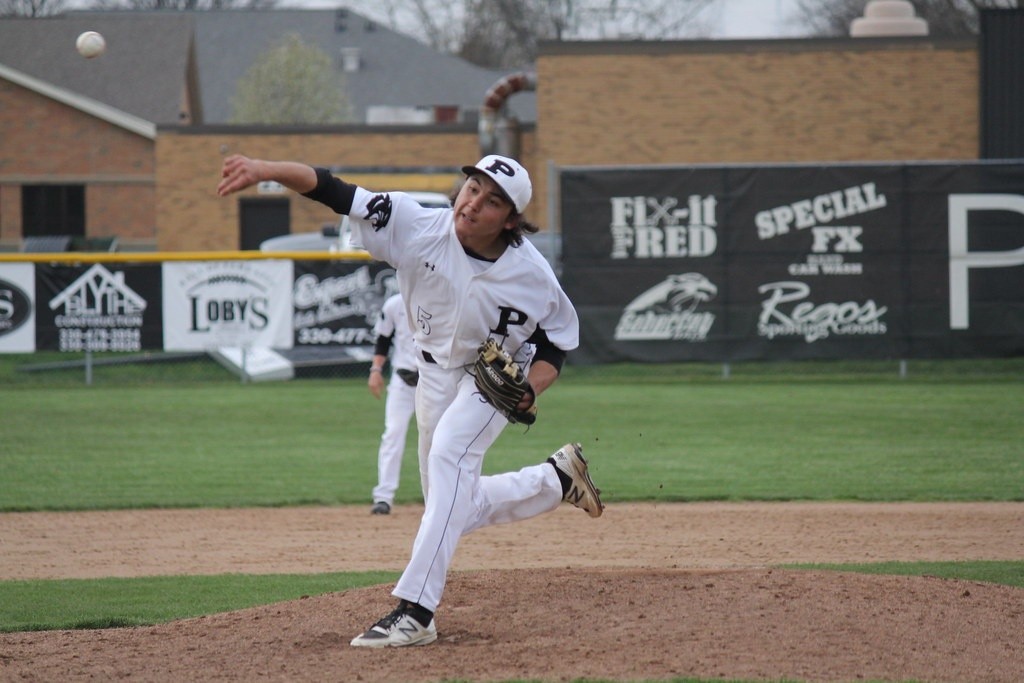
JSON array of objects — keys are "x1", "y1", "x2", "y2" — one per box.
[
  {"x1": 350, "y1": 606, "x2": 438, "y2": 649},
  {"x1": 547, "y1": 444, "x2": 604, "y2": 518}
]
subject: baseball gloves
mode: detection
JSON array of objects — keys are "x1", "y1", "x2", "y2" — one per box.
[{"x1": 463, "y1": 326, "x2": 538, "y2": 435}]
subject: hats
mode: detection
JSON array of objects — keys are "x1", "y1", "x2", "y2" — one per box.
[{"x1": 461, "y1": 155, "x2": 533, "y2": 214}]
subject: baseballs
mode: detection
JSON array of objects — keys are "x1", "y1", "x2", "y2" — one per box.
[{"x1": 76, "y1": 31, "x2": 107, "y2": 59}]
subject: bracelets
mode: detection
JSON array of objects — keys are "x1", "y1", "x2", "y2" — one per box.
[{"x1": 370, "y1": 367, "x2": 382, "y2": 373}]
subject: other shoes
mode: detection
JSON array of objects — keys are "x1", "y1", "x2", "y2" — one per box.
[{"x1": 370, "y1": 502, "x2": 389, "y2": 514}]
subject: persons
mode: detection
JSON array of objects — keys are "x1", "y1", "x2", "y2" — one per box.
[
  {"x1": 214, "y1": 150, "x2": 604, "y2": 650},
  {"x1": 367, "y1": 290, "x2": 421, "y2": 514}
]
